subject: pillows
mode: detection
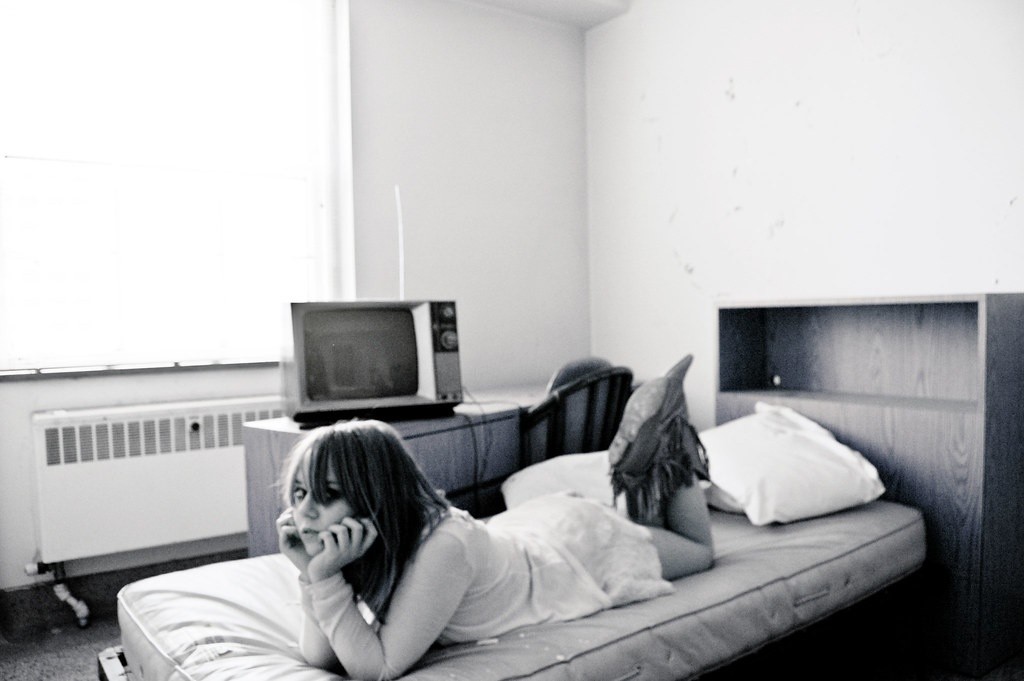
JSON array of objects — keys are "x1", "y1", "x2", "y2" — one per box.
[{"x1": 693, "y1": 402, "x2": 886, "y2": 527}]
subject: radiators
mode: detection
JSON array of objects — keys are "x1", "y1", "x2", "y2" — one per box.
[{"x1": 23, "y1": 392, "x2": 285, "y2": 630}]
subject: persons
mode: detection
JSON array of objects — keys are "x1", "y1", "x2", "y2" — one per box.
[{"x1": 275, "y1": 354, "x2": 714, "y2": 681}]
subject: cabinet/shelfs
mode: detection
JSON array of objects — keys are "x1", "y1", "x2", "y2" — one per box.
[
  {"x1": 242, "y1": 389, "x2": 522, "y2": 558},
  {"x1": 714, "y1": 291, "x2": 1023, "y2": 678}
]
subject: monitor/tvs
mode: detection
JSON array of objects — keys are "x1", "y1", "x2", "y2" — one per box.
[{"x1": 284, "y1": 301, "x2": 465, "y2": 428}]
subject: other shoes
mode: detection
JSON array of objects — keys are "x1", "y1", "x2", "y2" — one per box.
[{"x1": 612, "y1": 354, "x2": 711, "y2": 520}]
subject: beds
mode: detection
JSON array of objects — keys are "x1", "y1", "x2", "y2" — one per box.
[{"x1": 99, "y1": 491, "x2": 927, "y2": 681}]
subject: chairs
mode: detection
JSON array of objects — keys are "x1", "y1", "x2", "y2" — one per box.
[{"x1": 516, "y1": 357, "x2": 632, "y2": 474}]
